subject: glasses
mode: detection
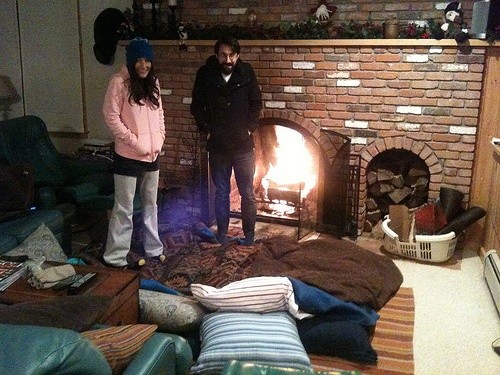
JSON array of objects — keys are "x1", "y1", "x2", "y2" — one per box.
[{"x1": 217, "y1": 52, "x2": 238, "y2": 59}]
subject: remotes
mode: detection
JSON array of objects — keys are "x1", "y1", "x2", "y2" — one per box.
[
  {"x1": 68, "y1": 272, "x2": 97, "y2": 294},
  {"x1": 52, "y1": 275, "x2": 83, "y2": 291}
]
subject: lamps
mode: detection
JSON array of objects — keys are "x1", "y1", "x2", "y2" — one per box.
[{"x1": 0, "y1": 77, "x2": 14, "y2": 120}]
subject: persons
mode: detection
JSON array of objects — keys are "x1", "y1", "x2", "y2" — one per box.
[
  {"x1": 102, "y1": 37, "x2": 166, "y2": 270},
  {"x1": 190, "y1": 36, "x2": 262, "y2": 245}
]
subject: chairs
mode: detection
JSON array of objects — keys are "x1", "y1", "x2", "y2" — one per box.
[
  {"x1": 0, "y1": 115, "x2": 141, "y2": 257},
  {"x1": 0, "y1": 324, "x2": 192, "y2": 375}
]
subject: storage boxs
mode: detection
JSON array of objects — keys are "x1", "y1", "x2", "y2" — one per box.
[{"x1": 383, "y1": 214, "x2": 456, "y2": 262}]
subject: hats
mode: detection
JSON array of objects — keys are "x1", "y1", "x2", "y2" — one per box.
[{"x1": 125, "y1": 37, "x2": 153, "y2": 64}]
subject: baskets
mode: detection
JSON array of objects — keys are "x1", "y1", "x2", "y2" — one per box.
[{"x1": 382, "y1": 214, "x2": 460, "y2": 262}]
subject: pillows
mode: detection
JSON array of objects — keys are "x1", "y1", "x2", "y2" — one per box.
[
  {"x1": 3, "y1": 223, "x2": 66, "y2": 260},
  {"x1": 78, "y1": 277, "x2": 378, "y2": 375}
]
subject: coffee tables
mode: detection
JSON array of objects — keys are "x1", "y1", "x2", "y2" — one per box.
[{"x1": 0, "y1": 261, "x2": 140, "y2": 333}]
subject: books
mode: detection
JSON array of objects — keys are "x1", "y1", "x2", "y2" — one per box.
[{"x1": 0, "y1": 260, "x2": 24, "y2": 291}]
